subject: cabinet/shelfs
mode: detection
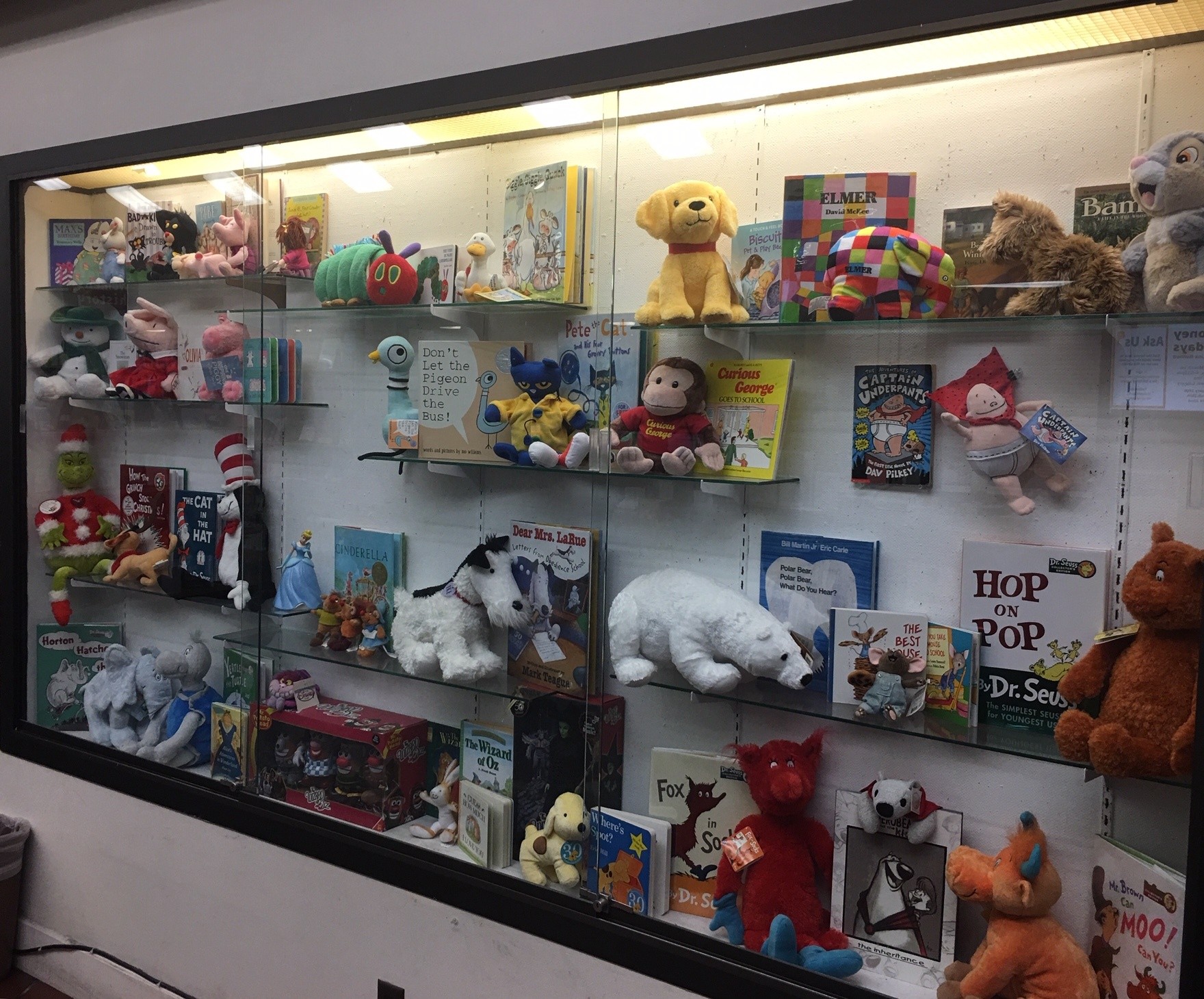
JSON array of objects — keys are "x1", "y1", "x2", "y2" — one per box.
[{"x1": 37, "y1": 241, "x2": 1200, "y2": 988}]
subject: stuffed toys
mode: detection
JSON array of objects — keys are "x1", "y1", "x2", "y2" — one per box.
[{"x1": 27, "y1": 129, "x2": 1202, "y2": 999}]
